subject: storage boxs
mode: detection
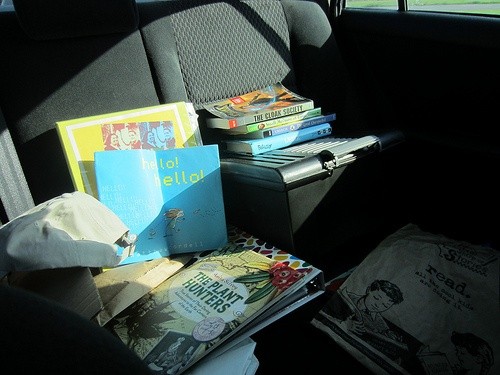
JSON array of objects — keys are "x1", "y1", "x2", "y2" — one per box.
[{"x1": 220, "y1": 130, "x2": 406, "y2": 263}]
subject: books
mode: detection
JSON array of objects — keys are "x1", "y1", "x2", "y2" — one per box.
[{"x1": 53, "y1": 81, "x2": 336, "y2": 375}]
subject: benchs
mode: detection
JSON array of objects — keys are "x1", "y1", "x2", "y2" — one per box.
[{"x1": 0, "y1": 0, "x2": 378, "y2": 375}]
